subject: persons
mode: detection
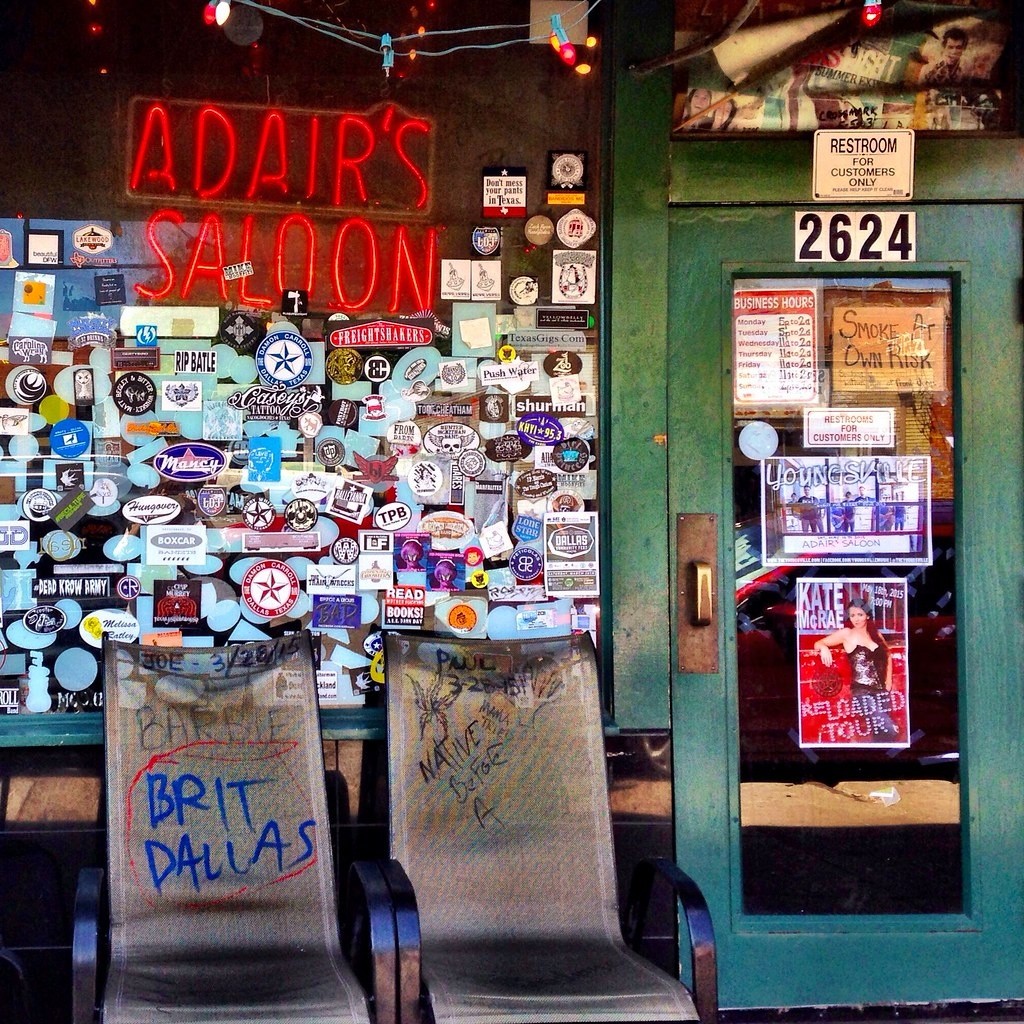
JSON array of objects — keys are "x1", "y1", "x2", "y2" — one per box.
[
  {"x1": 922, "y1": 28, "x2": 967, "y2": 79},
  {"x1": 680, "y1": 89, "x2": 737, "y2": 129},
  {"x1": 789, "y1": 485, "x2": 824, "y2": 532},
  {"x1": 874, "y1": 507, "x2": 906, "y2": 531},
  {"x1": 814, "y1": 598, "x2": 899, "y2": 743},
  {"x1": 840, "y1": 487, "x2": 872, "y2": 532}
]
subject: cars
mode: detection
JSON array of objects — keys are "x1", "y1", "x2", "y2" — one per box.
[{"x1": 734, "y1": 499, "x2": 958, "y2": 777}]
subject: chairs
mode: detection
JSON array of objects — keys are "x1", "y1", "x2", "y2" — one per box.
[
  {"x1": 378, "y1": 630, "x2": 719, "y2": 1024},
  {"x1": 73, "y1": 631, "x2": 398, "y2": 1024}
]
